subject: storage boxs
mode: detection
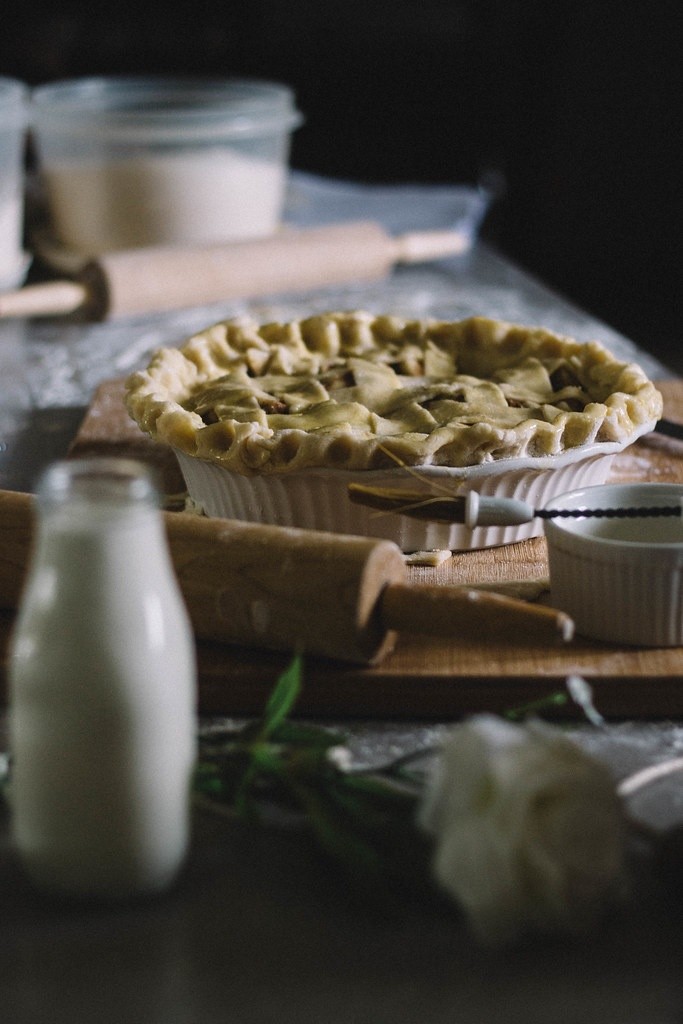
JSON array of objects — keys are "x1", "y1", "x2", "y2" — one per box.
[{"x1": 30, "y1": 78, "x2": 303, "y2": 254}]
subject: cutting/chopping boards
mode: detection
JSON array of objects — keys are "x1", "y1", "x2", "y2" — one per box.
[{"x1": 1, "y1": 238, "x2": 680, "y2": 722}]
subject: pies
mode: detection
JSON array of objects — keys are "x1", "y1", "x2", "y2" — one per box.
[{"x1": 123, "y1": 309, "x2": 664, "y2": 477}]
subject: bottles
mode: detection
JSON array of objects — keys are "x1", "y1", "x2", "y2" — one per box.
[{"x1": 4, "y1": 459, "x2": 200, "y2": 900}]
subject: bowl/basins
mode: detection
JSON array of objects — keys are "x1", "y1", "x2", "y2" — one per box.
[
  {"x1": 542, "y1": 482, "x2": 682, "y2": 646},
  {"x1": 1, "y1": 81, "x2": 34, "y2": 294},
  {"x1": 28, "y1": 77, "x2": 303, "y2": 280}
]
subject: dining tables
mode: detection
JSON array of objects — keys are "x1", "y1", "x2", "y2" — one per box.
[{"x1": 1, "y1": 165, "x2": 683, "y2": 1024}]
